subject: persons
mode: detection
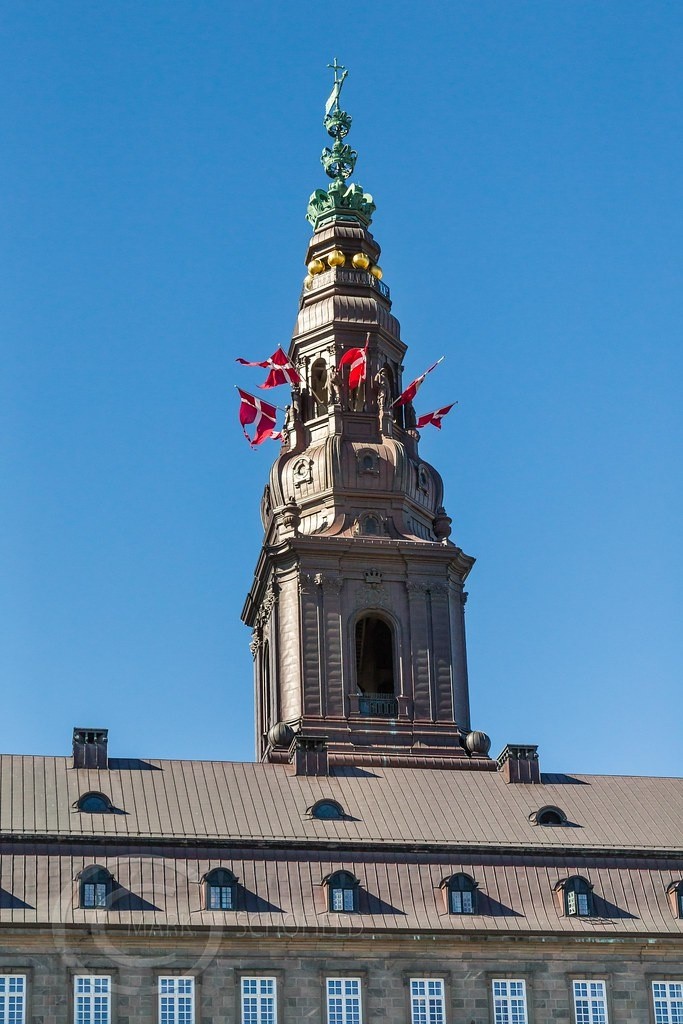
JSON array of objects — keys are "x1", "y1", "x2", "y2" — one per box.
[
  {"x1": 281, "y1": 404, "x2": 290, "y2": 445},
  {"x1": 374, "y1": 365, "x2": 391, "y2": 418},
  {"x1": 322, "y1": 365, "x2": 342, "y2": 405}
]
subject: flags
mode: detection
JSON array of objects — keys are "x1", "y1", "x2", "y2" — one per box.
[
  {"x1": 392, "y1": 362, "x2": 437, "y2": 408},
  {"x1": 414, "y1": 402, "x2": 456, "y2": 430},
  {"x1": 337, "y1": 334, "x2": 369, "y2": 390},
  {"x1": 235, "y1": 348, "x2": 302, "y2": 389},
  {"x1": 238, "y1": 389, "x2": 284, "y2": 451}
]
